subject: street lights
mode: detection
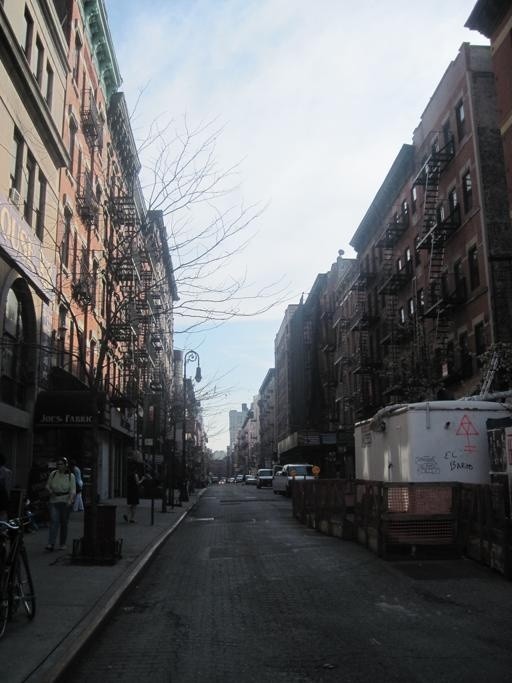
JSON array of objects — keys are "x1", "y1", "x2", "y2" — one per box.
[{"x1": 180, "y1": 348, "x2": 203, "y2": 502}]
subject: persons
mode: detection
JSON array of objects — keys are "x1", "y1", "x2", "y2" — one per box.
[
  {"x1": 0, "y1": 460, "x2": 13, "y2": 522},
  {"x1": 45, "y1": 456, "x2": 75, "y2": 551},
  {"x1": 123, "y1": 464, "x2": 148, "y2": 523},
  {"x1": 69, "y1": 459, "x2": 85, "y2": 512}
]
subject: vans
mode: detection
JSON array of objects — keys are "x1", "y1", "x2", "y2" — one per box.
[
  {"x1": 272, "y1": 463, "x2": 320, "y2": 497},
  {"x1": 255, "y1": 468, "x2": 277, "y2": 488}
]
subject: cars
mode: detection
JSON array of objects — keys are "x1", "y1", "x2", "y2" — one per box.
[{"x1": 218, "y1": 472, "x2": 257, "y2": 485}]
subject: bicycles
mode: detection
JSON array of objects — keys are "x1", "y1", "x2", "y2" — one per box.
[{"x1": 0, "y1": 508, "x2": 43, "y2": 639}]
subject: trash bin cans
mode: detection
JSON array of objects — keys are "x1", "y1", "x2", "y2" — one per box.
[{"x1": 84, "y1": 502, "x2": 117, "y2": 566}]
